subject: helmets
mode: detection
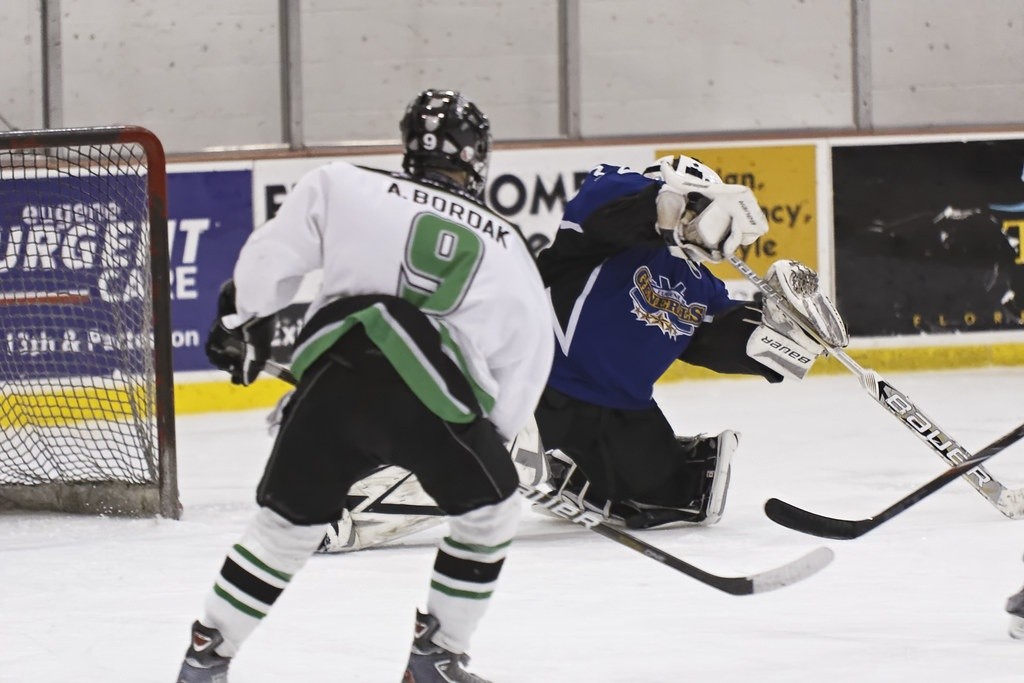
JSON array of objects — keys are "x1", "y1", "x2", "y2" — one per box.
[
  {"x1": 644, "y1": 155, "x2": 726, "y2": 266},
  {"x1": 399, "y1": 88, "x2": 489, "y2": 197}
]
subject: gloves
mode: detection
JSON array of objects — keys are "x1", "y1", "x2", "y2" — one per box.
[{"x1": 205, "y1": 278, "x2": 277, "y2": 387}]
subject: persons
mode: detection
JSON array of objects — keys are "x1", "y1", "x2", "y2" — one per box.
[
  {"x1": 177, "y1": 92, "x2": 556, "y2": 683},
  {"x1": 539, "y1": 155, "x2": 848, "y2": 529}
]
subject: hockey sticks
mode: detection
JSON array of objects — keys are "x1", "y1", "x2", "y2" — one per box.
[{"x1": 218, "y1": 248, "x2": 1023, "y2": 597}]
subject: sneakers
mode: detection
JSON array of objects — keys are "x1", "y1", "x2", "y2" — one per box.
[
  {"x1": 177, "y1": 620, "x2": 232, "y2": 683},
  {"x1": 1006, "y1": 588, "x2": 1024, "y2": 641},
  {"x1": 400, "y1": 607, "x2": 493, "y2": 683}
]
